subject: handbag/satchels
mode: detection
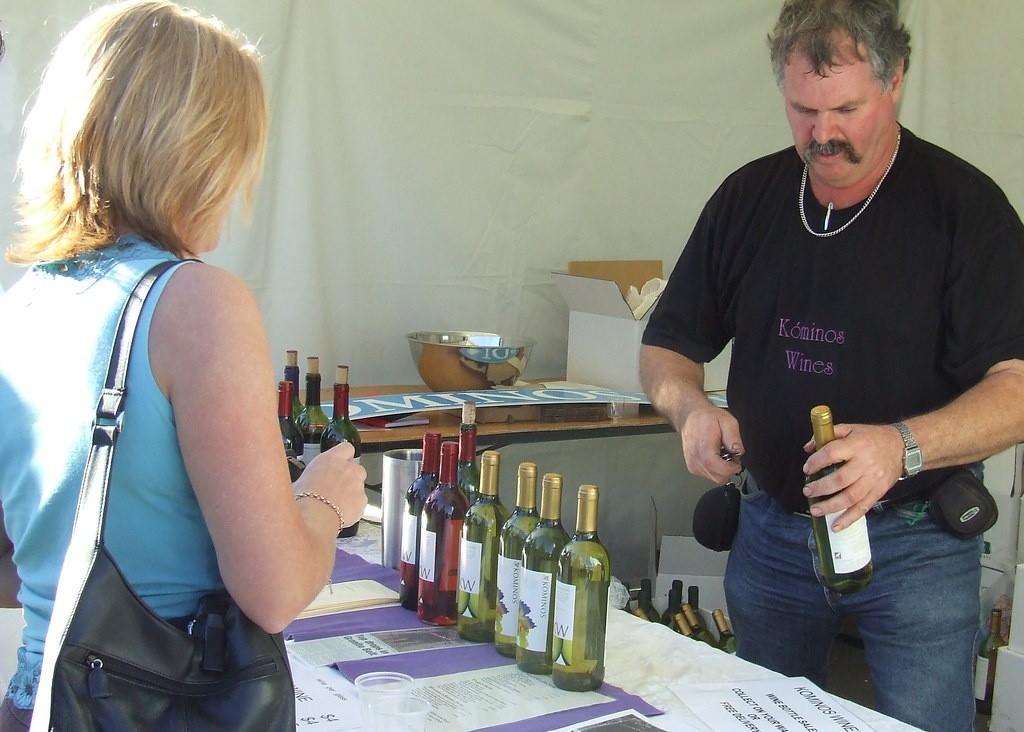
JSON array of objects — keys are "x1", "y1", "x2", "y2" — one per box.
[{"x1": 30, "y1": 257, "x2": 297, "y2": 732}]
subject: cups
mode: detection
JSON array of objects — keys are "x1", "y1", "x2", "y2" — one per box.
[
  {"x1": 368, "y1": 696, "x2": 431, "y2": 732},
  {"x1": 383, "y1": 449, "x2": 423, "y2": 569},
  {"x1": 355, "y1": 672, "x2": 415, "y2": 732}
]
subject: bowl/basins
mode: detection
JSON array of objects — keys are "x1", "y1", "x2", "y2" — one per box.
[{"x1": 406, "y1": 331, "x2": 538, "y2": 392}]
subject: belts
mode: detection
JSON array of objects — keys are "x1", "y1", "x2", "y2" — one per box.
[{"x1": 782, "y1": 484, "x2": 957, "y2": 516}]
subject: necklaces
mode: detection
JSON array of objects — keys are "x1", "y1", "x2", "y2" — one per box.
[{"x1": 799, "y1": 122, "x2": 904, "y2": 239}]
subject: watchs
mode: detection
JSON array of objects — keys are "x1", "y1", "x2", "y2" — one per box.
[{"x1": 886, "y1": 419, "x2": 923, "y2": 483}]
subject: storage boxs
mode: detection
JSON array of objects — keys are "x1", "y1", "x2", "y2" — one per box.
[
  {"x1": 436, "y1": 407, "x2": 543, "y2": 425},
  {"x1": 979, "y1": 566, "x2": 1009, "y2": 625},
  {"x1": 647, "y1": 496, "x2": 728, "y2": 616},
  {"x1": 550, "y1": 259, "x2": 733, "y2": 393},
  {"x1": 979, "y1": 443, "x2": 1024, "y2": 573},
  {"x1": 1007, "y1": 562, "x2": 1024, "y2": 656},
  {"x1": 629, "y1": 596, "x2": 736, "y2": 656},
  {"x1": 989, "y1": 643, "x2": 1024, "y2": 732}
]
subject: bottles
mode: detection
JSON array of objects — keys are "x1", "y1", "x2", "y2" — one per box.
[
  {"x1": 418, "y1": 442, "x2": 470, "y2": 626},
  {"x1": 458, "y1": 451, "x2": 510, "y2": 642},
  {"x1": 806, "y1": 406, "x2": 873, "y2": 594},
  {"x1": 495, "y1": 463, "x2": 540, "y2": 658},
  {"x1": 320, "y1": 365, "x2": 362, "y2": 539},
  {"x1": 284, "y1": 350, "x2": 304, "y2": 422},
  {"x1": 712, "y1": 609, "x2": 736, "y2": 657},
  {"x1": 552, "y1": 485, "x2": 610, "y2": 692},
  {"x1": 974, "y1": 609, "x2": 1005, "y2": 716},
  {"x1": 400, "y1": 433, "x2": 442, "y2": 612},
  {"x1": 662, "y1": 580, "x2": 717, "y2": 649},
  {"x1": 278, "y1": 381, "x2": 306, "y2": 484},
  {"x1": 458, "y1": 403, "x2": 480, "y2": 507},
  {"x1": 517, "y1": 473, "x2": 571, "y2": 674},
  {"x1": 619, "y1": 579, "x2": 660, "y2": 624},
  {"x1": 294, "y1": 357, "x2": 330, "y2": 467}
]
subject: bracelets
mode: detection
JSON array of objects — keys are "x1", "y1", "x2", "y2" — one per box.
[{"x1": 291, "y1": 491, "x2": 344, "y2": 537}]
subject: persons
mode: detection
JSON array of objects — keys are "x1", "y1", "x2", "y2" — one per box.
[
  {"x1": 0, "y1": 0, "x2": 367, "y2": 732},
  {"x1": 638, "y1": 0, "x2": 1024, "y2": 732}
]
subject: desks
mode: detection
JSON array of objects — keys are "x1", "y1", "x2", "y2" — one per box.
[
  {"x1": 283, "y1": 518, "x2": 923, "y2": 732},
  {"x1": 298, "y1": 379, "x2": 677, "y2": 495}
]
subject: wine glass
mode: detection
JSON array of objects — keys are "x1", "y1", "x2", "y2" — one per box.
[{"x1": 606, "y1": 397, "x2": 623, "y2": 424}]
